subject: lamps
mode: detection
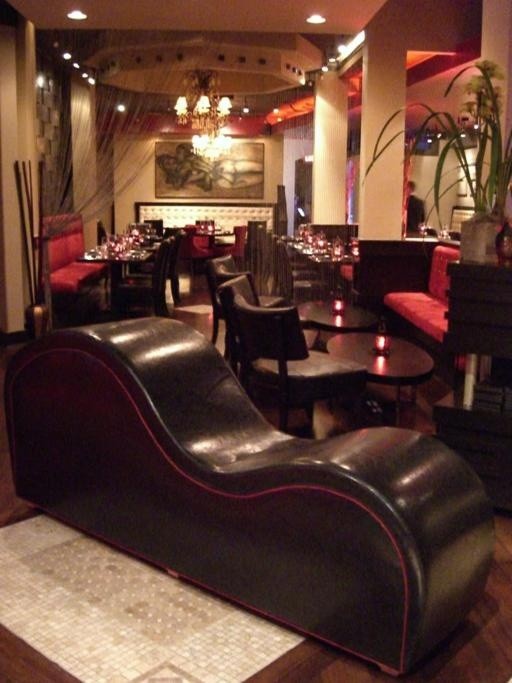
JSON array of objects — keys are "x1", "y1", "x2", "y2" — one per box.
[{"x1": 165, "y1": 67, "x2": 237, "y2": 132}]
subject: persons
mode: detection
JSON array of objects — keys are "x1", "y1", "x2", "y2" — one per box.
[{"x1": 406, "y1": 180, "x2": 425, "y2": 237}]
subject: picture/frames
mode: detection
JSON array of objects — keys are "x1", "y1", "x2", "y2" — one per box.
[{"x1": 152, "y1": 140, "x2": 267, "y2": 201}]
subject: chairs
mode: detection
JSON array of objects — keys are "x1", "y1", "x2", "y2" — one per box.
[{"x1": 1, "y1": 200, "x2": 511, "y2": 443}]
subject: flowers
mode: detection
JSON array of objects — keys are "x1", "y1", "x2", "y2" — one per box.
[{"x1": 350, "y1": 57, "x2": 512, "y2": 221}]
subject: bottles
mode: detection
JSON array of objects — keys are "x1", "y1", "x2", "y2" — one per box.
[{"x1": 495, "y1": 221, "x2": 511, "y2": 267}]
